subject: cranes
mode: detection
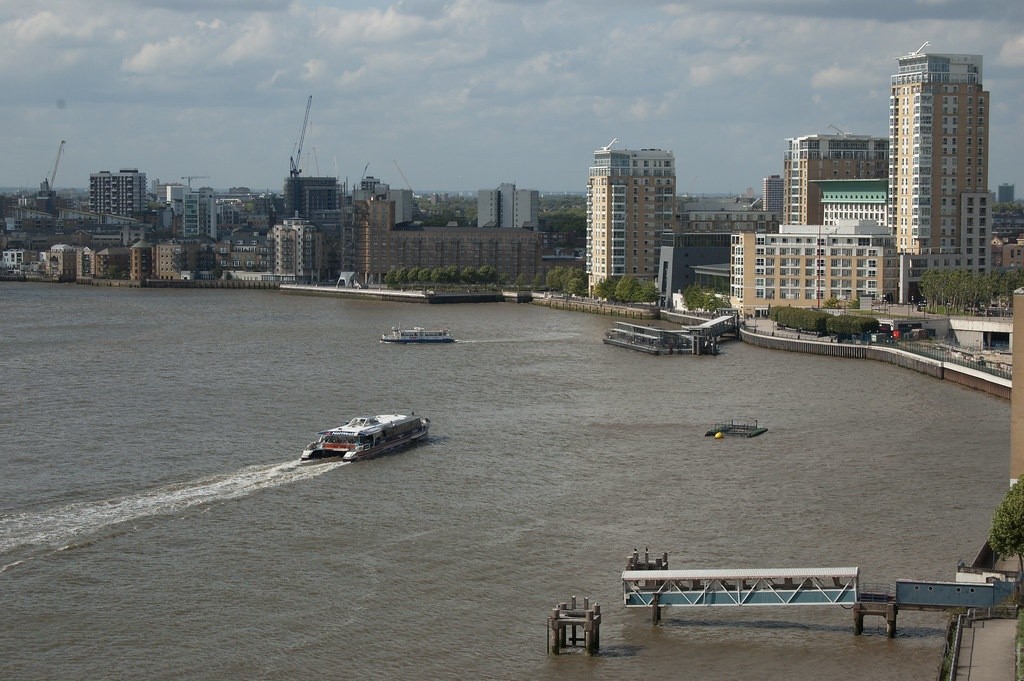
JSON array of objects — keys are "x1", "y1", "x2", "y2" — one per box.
[
  {"x1": 359, "y1": 162, "x2": 369, "y2": 189},
  {"x1": 289, "y1": 95, "x2": 312, "y2": 218},
  {"x1": 393, "y1": 159, "x2": 412, "y2": 190},
  {"x1": 46, "y1": 140, "x2": 66, "y2": 197},
  {"x1": 182, "y1": 176, "x2": 209, "y2": 187}
]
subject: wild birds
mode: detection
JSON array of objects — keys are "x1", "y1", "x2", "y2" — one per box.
[
  {"x1": 646, "y1": 547, "x2": 649, "y2": 552},
  {"x1": 633, "y1": 547, "x2": 637, "y2": 552}
]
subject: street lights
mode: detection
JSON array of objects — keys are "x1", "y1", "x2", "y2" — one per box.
[
  {"x1": 884, "y1": 299, "x2": 886, "y2": 313},
  {"x1": 871, "y1": 301, "x2": 873, "y2": 316},
  {"x1": 844, "y1": 299, "x2": 846, "y2": 313},
  {"x1": 912, "y1": 296, "x2": 914, "y2": 312},
  {"x1": 924, "y1": 300, "x2": 926, "y2": 318}
]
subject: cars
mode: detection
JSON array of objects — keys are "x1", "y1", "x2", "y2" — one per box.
[{"x1": 918, "y1": 301, "x2": 926, "y2": 307}]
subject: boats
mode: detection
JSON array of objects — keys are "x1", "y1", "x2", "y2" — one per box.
[
  {"x1": 380, "y1": 327, "x2": 455, "y2": 343},
  {"x1": 300, "y1": 412, "x2": 431, "y2": 464}
]
